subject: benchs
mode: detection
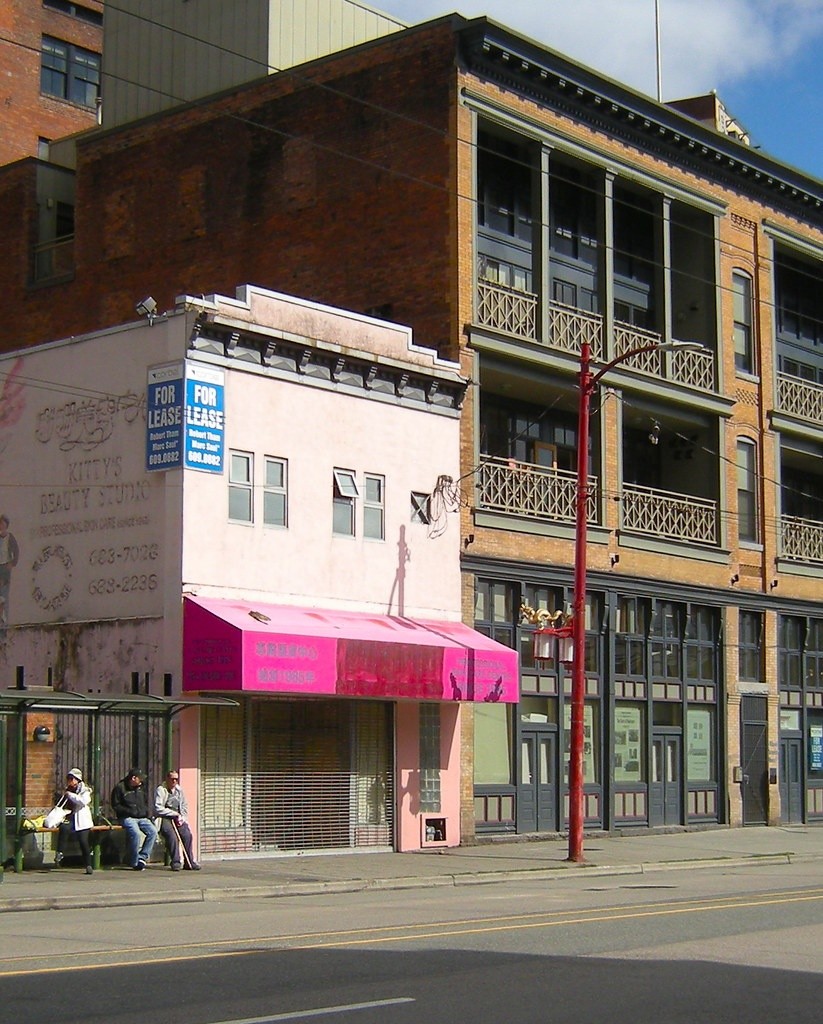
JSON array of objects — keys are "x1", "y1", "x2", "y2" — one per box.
[{"x1": 17, "y1": 825, "x2": 172, "y2": 870}]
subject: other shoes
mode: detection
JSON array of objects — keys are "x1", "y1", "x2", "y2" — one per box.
[
  {"x1": 86, "y1": 866, "x2": 92, "y2": 874},
  {"x1": 173, "y1": 862, "x2": 181, "y2": 870},
  {"x1": 137, "y1": 867, "x2": 145, "y2": 871},
  {"x1": 138, "y1": 858, "x2": 146, "y2": 867},
  {"x1": 183, "y1": 863, "x2": 201, "y2": 870},
  {"x1": 54, "y1": 853, "x2": 64, "y2": 861}
]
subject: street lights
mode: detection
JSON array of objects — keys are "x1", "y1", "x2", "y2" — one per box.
[{"x1": 564, "y1": 338, "x2": 704, "y2": 861}]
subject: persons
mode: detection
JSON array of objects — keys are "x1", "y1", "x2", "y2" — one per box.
[
  {"x1": 153, "y1": 771, "x2": 202, "y2": 871},
  {"x1": 112, "y1": 768, "x2": 158, "y2": 869},
  {"x1": 54, "y1": 767, "x2": 93, "y2": 875}
]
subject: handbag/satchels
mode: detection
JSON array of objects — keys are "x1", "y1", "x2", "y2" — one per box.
[{"x1": 44, "y1": 794, "x2": 68, "y2": 828}]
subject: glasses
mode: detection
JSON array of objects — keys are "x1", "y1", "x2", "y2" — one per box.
[
  {"x1": 67, "y1": 776, "x2": 74, "y2": 780},
  {"x1": 170, "y1": 778, "x2": 179, "y2": 781}
]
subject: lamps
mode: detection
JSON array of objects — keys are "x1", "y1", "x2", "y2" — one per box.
[
  {"x1": 34, "y1": 724, "x2": 50, "y2": 742},
  {"x1": 465, "y1": 534, "x2": 474, "y2": 546},
  {"x1": 135, "y1": 298, "x2": 158, "y2": 320},
  {"x1": 770, "y1": 580, "x2": 778, "y2": 589},
  {"x1": 648, "y1": 426, "x2": 660, "y2": 445},
  {"x1": 731, "y1": 574, "x2": 739, "y2": 586},
  {"x1": 589, "y1": 406, "x2": 598, "y2": 416},
  {"x1": 610, "y1": 553, "x2": 619, "y2": 566}
]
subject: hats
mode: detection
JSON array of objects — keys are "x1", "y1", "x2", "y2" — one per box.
[
  {"x1": 129, "y1": 768, "x2": 147, "y2": 778},
  {"x1": 68, "y1": 768, "x2": 83, "y2": 781}
]
